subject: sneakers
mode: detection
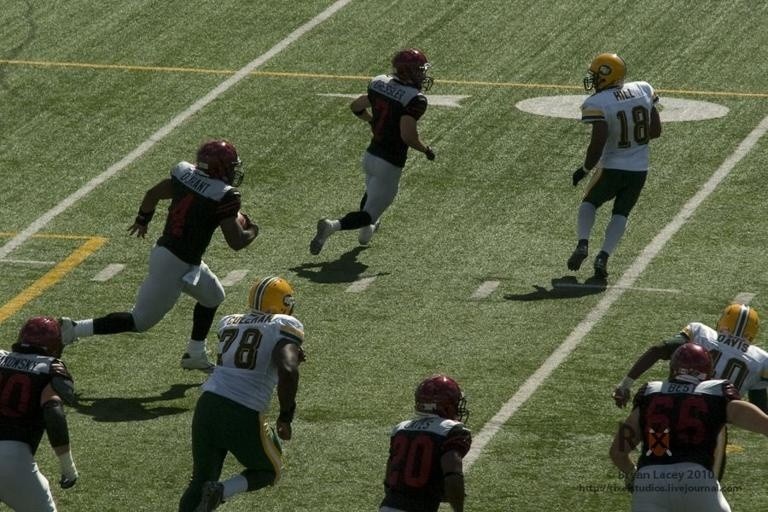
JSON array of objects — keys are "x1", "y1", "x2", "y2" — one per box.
[
  {"x1": 180, "y1": 352, "x2": 216, "y2": 376},
  {"x1": 194, "y1": 481, "x2": 226, "y2": 511},
  {"x1": 566, "y1": 246, "x2": 588, "y2": 273},
  {"x1": 310, "y1": 217, "x2": 332, "y2": 256},
  {"x1": 60, "y1": 315, "x2": 81, "y2": 348},
  {"x1": 358, "y1": 224, "x2": 375, "y2": 245},
  {"x1": 593, "y1": 259, "x2": 608, "y2": 279}
]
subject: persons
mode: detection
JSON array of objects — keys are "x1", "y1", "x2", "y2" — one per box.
[
  {"x1": 567, "y1": 53, "x2": 661, "y2": 278},
  {"x1": 611, "y1": 304, "x2": 768, "y2": 422},
  {"x1": 59, "y1": 139, "x2": 259, "y2": 372},
  {"x1": 609, "y1": 342, "x2": 768, "y2": 512},
  {"x1": 178, "y1": 276, "x2": 307, "y2": 511},
  {"x1": 0, "y1": 316, "x2": 80, "y2": 512},
  {"x1": 310, "y1": 47, "x2": 435, "y2": 255},
  {"x1": 379, "y1": 373, "x2": 472, "y2": 512}
]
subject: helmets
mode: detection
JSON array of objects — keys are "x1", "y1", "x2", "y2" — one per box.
[
  {"x1": 196, "y1": 141, "x2": 246, "y2": 187},
  {"x1": 391, "y1": 49, "x2": 435, "y2": 97},
  {"x1": 414, "y1": 375, "x2": 470, "y2": 425},
  {"x1": 582, "y1": 53, "x2": 627, "y2": 92},
  {"x1": 16, "y1": 317, "x2": 64, "y2": 356},
  {"x1": 717, "y1": 303, "x2": 761, "y2": 344},
  {"x1": 671, "y1": 344, "x2": 718, "y2": 382},
  {"x1": 248, "y1": 275, "x2": 296, "y2": 316}
]
